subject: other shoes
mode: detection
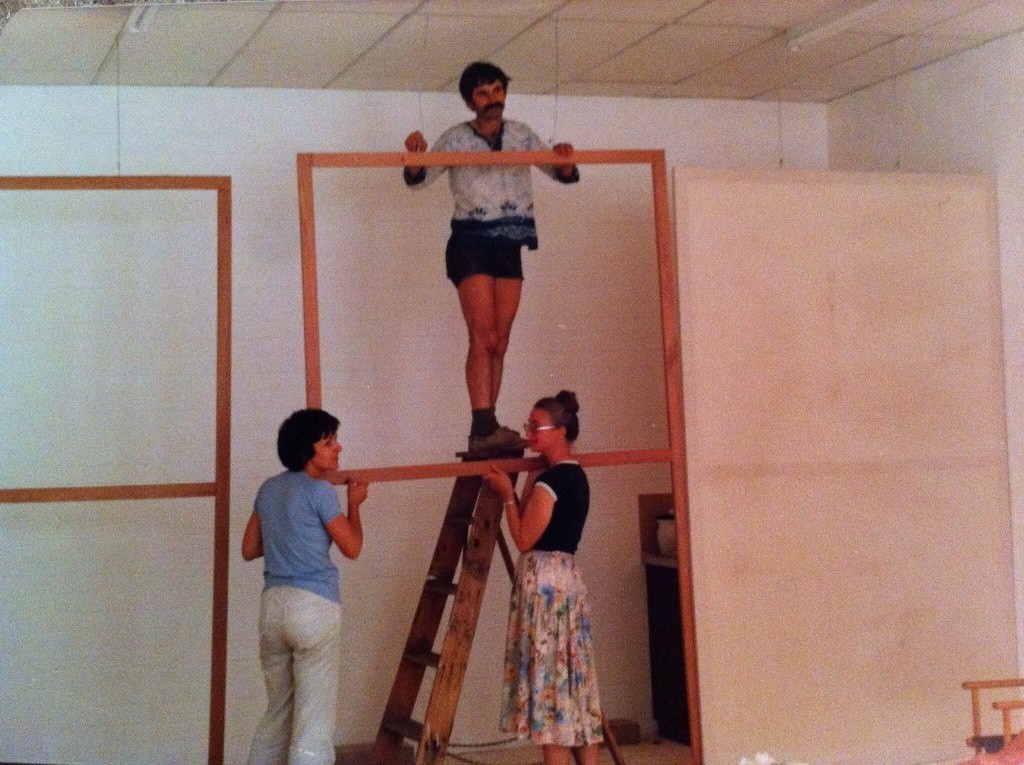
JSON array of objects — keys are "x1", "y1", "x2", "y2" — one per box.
[{"x1": 466, "y1": 423, "x2": 535, "y2": 455}]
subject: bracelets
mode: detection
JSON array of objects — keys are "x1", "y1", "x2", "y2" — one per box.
[{"x1": 504, "y1": 501, "x2": 515, "y2": 505}]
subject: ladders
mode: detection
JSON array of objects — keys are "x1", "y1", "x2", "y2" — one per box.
[{"x1": 371, "y1": 442, "x2": 626, "y2": 765}]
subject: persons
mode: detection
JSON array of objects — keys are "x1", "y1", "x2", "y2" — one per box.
[
  {"x1": 241, "y1": 408, "x2": 370, "y2": 765},
  {"x1": 403, "y1": 61, "x2": 579, "y2": 450},
  {"x1": 482, "y1": 391, "x2": 605, "y2": 765}
]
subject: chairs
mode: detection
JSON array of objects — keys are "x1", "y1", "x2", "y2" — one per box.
[{"x1": 960, "y1": 676, "x2": 1024, "y2": 765}]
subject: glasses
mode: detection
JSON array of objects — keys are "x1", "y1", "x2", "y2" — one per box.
[{"x1": 522, "y1": 421, "x2": 560, "y2": 435}]
